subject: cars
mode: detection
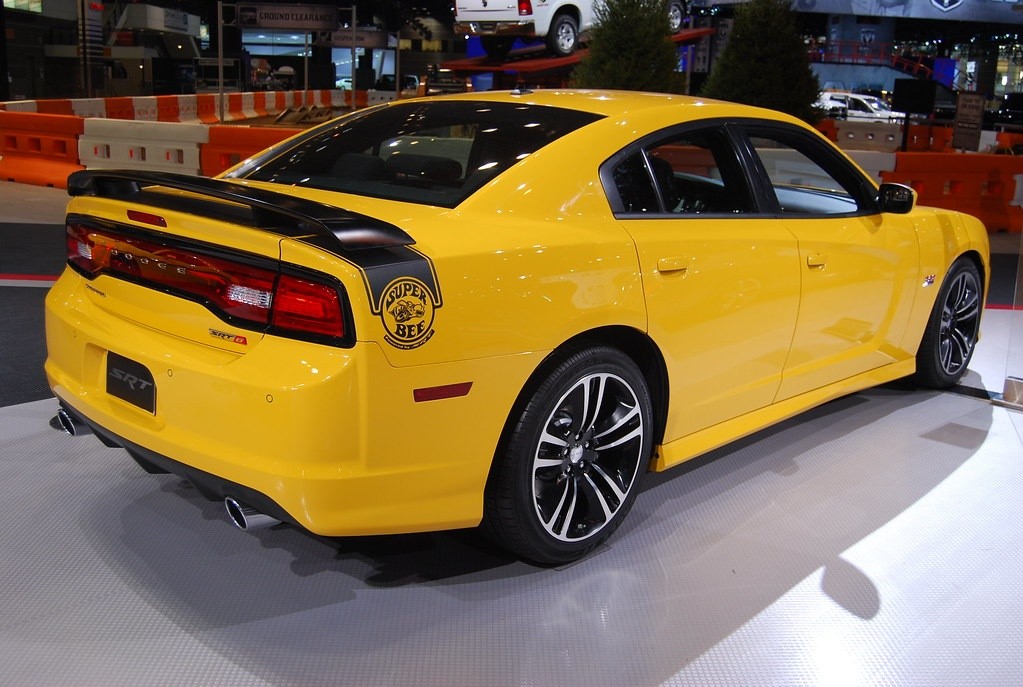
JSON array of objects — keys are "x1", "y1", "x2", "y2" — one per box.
[
  {"x1": 336, "y1": 78, "x2": 353, "y2": 90},
  {"x1": 366, "y1": 59, "x2": 466, "y2": 107},
  {"x1": 43, "y1": 88, "x2": 990, "y2": 569}
]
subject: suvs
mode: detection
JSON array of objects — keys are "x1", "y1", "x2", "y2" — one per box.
[
  {"x1": 812, "y1": 91, "x2": 957, "y2": 123},
  {"x1": 451, "y1": 0, "x2": 685, "y2": 56}
]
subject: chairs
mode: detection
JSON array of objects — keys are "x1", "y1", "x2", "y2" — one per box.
[
  {"x1": 377, "y1": 155, "x2": 464, "y2": 203},
  {"x1": 619, "y1": 155, "x2": 680, "y2": 213}
]
